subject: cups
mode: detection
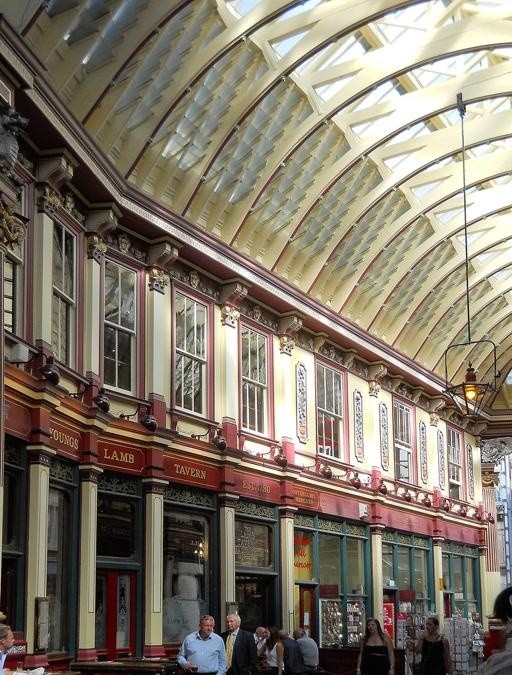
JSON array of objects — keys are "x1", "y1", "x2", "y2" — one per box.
[{"x1": 190, "y1": 666, "x2": 198, "y2": 673}]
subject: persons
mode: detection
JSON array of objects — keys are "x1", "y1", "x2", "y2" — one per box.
[
  {"x1": 477, "y1": 587, "x2": 512, "y2": 675},
  {"x1": 356, "y1": 617, "x2": 395, "y2": 675},
  {"x1": 416, "y1": 616, "x2": 454, "y2": 675},
  {"x1": 0, "y1": 623, "x2": 15, "y2": 675},
  {"x1": 177, "y1": 612, "x2": 320, "y2": 675}
]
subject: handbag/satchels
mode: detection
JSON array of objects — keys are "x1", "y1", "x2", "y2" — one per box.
[
  {"x1": 256, "y1": 660, "x2": 269, "y2": 672},
  {"x1": 412, "y1": 663, "x2": 424, "y2": 675}
]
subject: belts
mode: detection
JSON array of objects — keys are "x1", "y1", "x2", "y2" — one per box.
[{"x1": 304, "y1": 665, "x2": 318, "y2": 669}]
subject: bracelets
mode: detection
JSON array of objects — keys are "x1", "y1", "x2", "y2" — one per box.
[
  {"x1": 356, "y1": 665, "x2": 361, "y2": 669},
  {"x1": 390, "y1": 666, "x2": 395, "y2": 669}
]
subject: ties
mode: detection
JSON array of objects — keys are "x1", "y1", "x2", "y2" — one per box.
[{"x1": 227, "y1": 633, "x2": 233, "y2": 667}]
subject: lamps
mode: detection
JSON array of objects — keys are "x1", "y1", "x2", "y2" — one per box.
[
  {"x1": 441, "y1": 91, "x2": 501, "y2": 419},
  {"x1": 12, "y1": 352, "x2": 61, "y2": 385},
  {"x1": 258, "y1": 444, "x2": 364, "y2": 493},
  {"x1": 368, "y1": 475, "x2": 495, "y2": 525},
  {"x1": 68, "y1": 383, "x2": 157, "y2": 433},
  {"x1": 191, "y1": 426, "x2": 227, "y2": 452}
]
